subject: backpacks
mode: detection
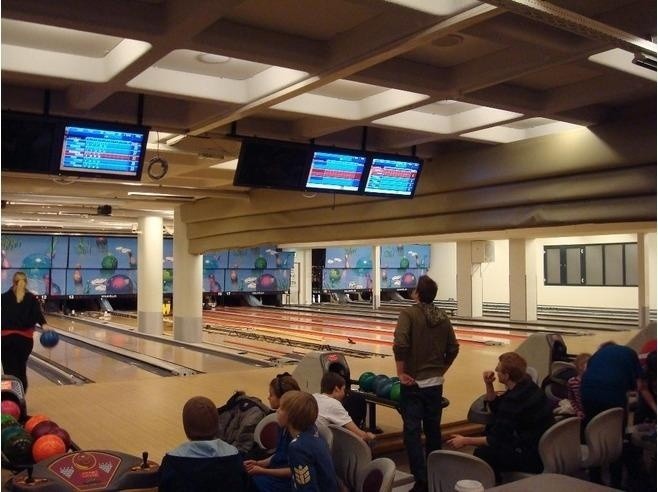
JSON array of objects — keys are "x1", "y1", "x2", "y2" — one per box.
[{"x1": 217, "y1": 390, "x2": 272, "y2": 457}]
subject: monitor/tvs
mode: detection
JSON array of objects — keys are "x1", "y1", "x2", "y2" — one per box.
[
  {"x1": 2, "y1": 108, "x2": 61, "y2": 173},
  {"x1": 57, "y1": 114, "x2": 149, "y2": 181},
  {"x1": 362, "y1": 152, "x2": 422, "y2": 199},
  {"x1": 305, "y1": 146, "x2": 368, "y2": 195},
  {"x1": 232, "y1": 135, "x2": 309, "y2": 192}
]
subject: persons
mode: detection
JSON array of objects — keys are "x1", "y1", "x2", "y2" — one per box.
[
  {"x1": 242, "y1": 370, "x2": 302, "y2": 491},
  {"x1": 567, "y1": 338, "x2": 657, "y2": 492},
  {"x1": 274, "y1": 389, "x2": 339, "y2": 491},
  {"x1": 309, "y1": 372, "x2": 376, "y2": 448},
  {"x1": 391, "y1": 274, "x2": 459, "y2": 492},
  {"x1": 155, "y1": 396, "x2": 259, "y2": 490},
  {"x1": 446, "y1": 351, "x2": 557, "y2": 482},
  {"x1": 1, "y1": 270, "x2": 49, "y2": 402}
]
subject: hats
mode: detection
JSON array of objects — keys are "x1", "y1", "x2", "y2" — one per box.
[{"x1": 183, "y1": 396, "x2": 219, "y2": 438}]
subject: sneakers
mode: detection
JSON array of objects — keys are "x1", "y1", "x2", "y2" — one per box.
[
  {"x1": 408, "y1": 483, "x2": 430, "y2": 491},
  {"x1": 591, "y1": 480, "x2": 632, "y2": 492}
]
